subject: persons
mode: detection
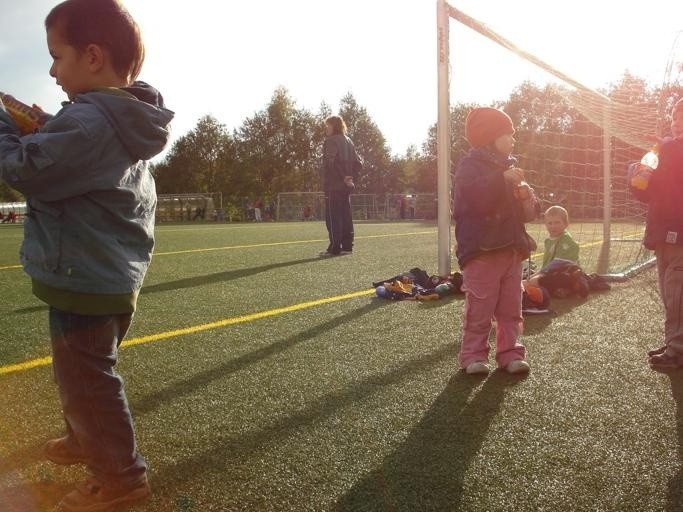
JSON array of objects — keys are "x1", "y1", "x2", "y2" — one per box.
[
  {"x1": 193, "y1": 204, "x2": 205, "y2": 221},
  {"x1": 318, "y1": 115, "x2": 360, "y2": 257},
  {"x1": 448, "y1": 106, "x2": 551, "y2": 382},
  {"x1": 0, "y1": 1, "x2": 176, "y2": 512},
  {"x1": 399, "y1": 195, "x2": 406, "y2": 220},
  {"x1": 629, "y1": 99, "x2": 683, "y2": 368},
  {"x1": 541, "y1": 205, "x2": 578, "y2": 271},
  {"x1": 245, "y1": 196, "x2": 275, "y2": 222},
  {"x1": 410, "y1": 195, "x2": 417, "y2": 218},
  {"x1": 316, "y1": 197, "x2": 322, "y2": 220},
  {"x1": 0, "y1": 211, "x2": 18, "y2": 223}
]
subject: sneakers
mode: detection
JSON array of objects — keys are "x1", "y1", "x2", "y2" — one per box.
[
  {"x1": 51, "y1": 470, "x2": 151, "y2": 512},
  {"x1": 504, "y1": 358, "x2": 531, "y2": 374},
  {"x1": 42, "y1": 434, "x2": 104, "y2": 470},
  {"x1": 317, "y1": 248, "x2": 352, "y2": 257},
  {"x1": 464, "y1": 360, "x2": 492, "y2": 375},
  {"x1": 646, "y1": 344, "x2": 682, "y2": 367}
]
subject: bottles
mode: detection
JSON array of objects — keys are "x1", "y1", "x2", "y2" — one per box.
[
  {"x1": 633, "y1": 145, "x2": 660, "y2": 190},
  {"x1": 0, "y1": 92, "x2": 54, "y2": 137},
  {"x1": 376, "y1": 286, "x2": 399, "y2": 301},
  {"x1": 517, "y1": 182, "x2": 536, "y2": 221}
]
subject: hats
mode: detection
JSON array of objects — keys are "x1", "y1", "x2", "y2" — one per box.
[{"x1": 464, "y1": 108, "x2": 513, "y2": 152}]
